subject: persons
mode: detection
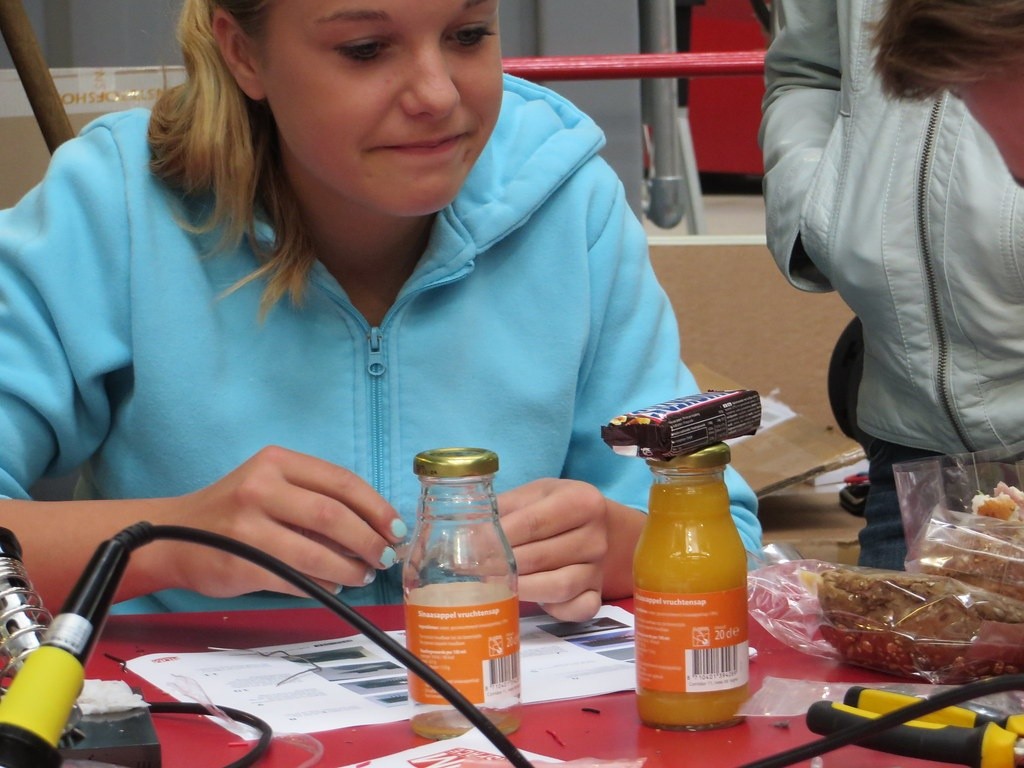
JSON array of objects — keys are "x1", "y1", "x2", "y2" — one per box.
[
  {"x1": 750, "y1": 0, "x2": 1024, "y2": 563},
  {"x1": 867, "y1": 0, "x2": 1024, "y2": 188},
  {"x1": 0, "y1": 2, "x2": 764, "y2": 626}
]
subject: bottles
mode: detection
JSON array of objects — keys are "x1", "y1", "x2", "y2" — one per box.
[
  {"x1": 403, "y1": 446, "x2": 521, "y2": 742},
  {"x1": 630, "y1": 440, "x2": 751, "y2": 733}
]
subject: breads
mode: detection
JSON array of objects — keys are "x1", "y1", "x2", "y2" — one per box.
[{"x1": 807, "y1": 479, "x2": 1024, "y2": 686}]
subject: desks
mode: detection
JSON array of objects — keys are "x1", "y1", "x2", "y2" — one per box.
[{"x1": 1, "y1": 586, "x2": 1024, "y2": 767}]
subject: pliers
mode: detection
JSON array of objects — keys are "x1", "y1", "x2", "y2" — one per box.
[{"x1": 805, "y1": 684, "x2": 1024, "y2": 768}]
